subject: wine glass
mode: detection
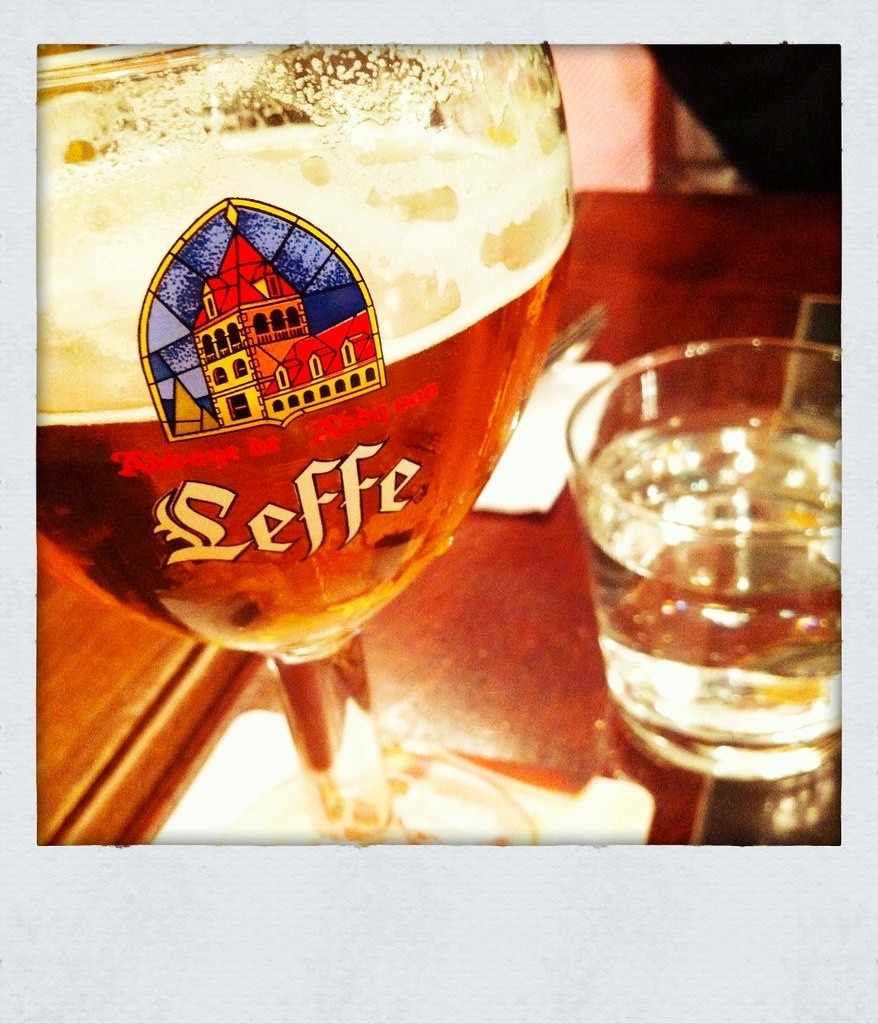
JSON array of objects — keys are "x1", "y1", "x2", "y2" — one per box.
[{"x1": 37, "y1": 45, "x2": 574, "y2": 844}]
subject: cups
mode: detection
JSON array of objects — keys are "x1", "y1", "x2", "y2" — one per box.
[{"x1": 565, "y1": 337, "x2": 844, "y2": 781}]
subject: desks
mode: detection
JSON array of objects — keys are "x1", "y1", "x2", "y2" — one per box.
[{"x1": 38, "y1": 189, "x2": 841, "y2": 846}]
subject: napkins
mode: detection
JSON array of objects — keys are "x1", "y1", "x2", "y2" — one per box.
[{"x1": 471, "y1": 361, "x2": 621, "y2": 516}]
